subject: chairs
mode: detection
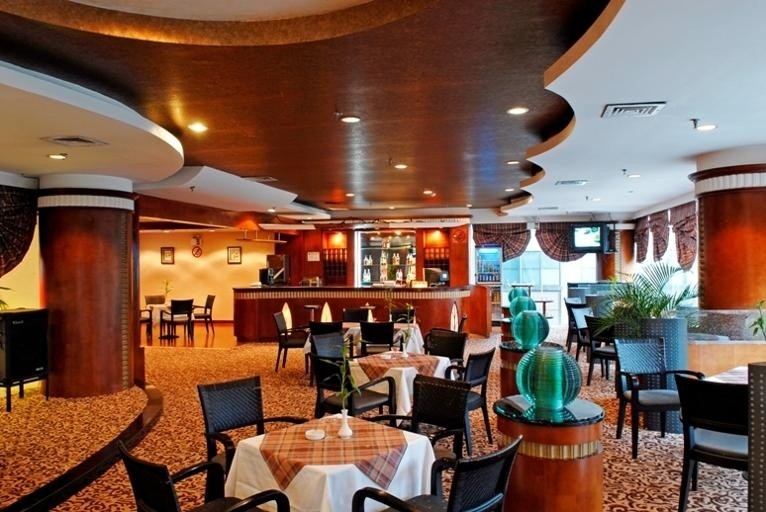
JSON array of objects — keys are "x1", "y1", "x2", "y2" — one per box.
[
  {"x1": 117, "y1": 437, "x2": 290, "y2": 511},
  {"x1": 140, "y1": 295, "x2": 215, "y2": 345},
  {"x1": 196, "y1": 374, "x2": 310, "y2": 478},
  {"x1": 613, "y1": 339, "x2": 705, "y2": 458},
  {"x1": 351, "y1": 433, "x2": 525, "y2": 512},
  {"x1": 670, "y1": 372, "x2": 753, "y2": 512}
]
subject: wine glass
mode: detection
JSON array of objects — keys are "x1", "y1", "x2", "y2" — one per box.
[{"x1": 390, "y1": 346, "x2": 400, "y2": 361}]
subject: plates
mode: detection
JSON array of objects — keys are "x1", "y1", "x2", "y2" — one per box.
[
  {"x1": 384, "y1": 280, "x2": 396, "y2": 288},
  {"x1": 411, "y1": 281, "x2": 428, "y2": 289}
]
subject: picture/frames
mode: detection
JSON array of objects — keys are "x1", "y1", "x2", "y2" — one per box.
[
  {"x1": 161, "y1": 246, "x2": 174, "y2": 264},
  {"x1": 227, "y1": 245, "x2": 241, "y2": 265}
]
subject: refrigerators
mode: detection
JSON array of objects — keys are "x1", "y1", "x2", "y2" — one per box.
[{"x1": 474, "y1": 243, "x2": 503, "y2": 326}]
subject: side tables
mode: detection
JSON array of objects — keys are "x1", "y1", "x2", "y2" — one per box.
[{"x1": 493, "y1": 392, "x2": 605, "y2": 512}]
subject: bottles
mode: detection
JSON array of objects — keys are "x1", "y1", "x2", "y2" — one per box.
[
  {"x1": 363, "y1": 255, "x2": 369, "y2": 265},
  {"x1": 399, "y1": 269, "x2": 403, "y2": 280},
  {"x1": 406, "y1": 253, "x2": 416, "y2": 265},
  {"x1": 384, "y1": 252, "x2": 387, "y2": 266},
  {"x1": 380, "y1": 250, "x2": 384, "y2": 265},
  {"x1": 362, "y1": 269, "x2": 367, "y2": 282},
  {"x1": 392, "y1": 253, "x2": 396, "y2": 265},
  {"x1": 367, "y1": 269, "x2": 371, "y2": 282},
  {"x1": 380, "y1": 272, "x2": 384, "y2": 284},
  {"x1": 384, "y1": 273, "x2": 387, "y2": 282},
  {"x1": 396, "y1": 253, "x2": 401, "y2": 265},
  {"x1": 368, "y1": 255, "x2": 373, "y2": 265},
  {"x1": 396, "y1": 270, "x2": 400, "y2": 280}
]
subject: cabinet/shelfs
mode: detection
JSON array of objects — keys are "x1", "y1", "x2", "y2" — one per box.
[
  {"x1": 0, "y1": 308, "x2": 50, "y2": 412},
  {"x1": 360, "y1": 230, "x2": 416, "y2": 286}
]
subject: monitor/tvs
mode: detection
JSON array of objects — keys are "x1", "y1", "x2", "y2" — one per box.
[
  {"x1": 423, "y1": 267, "x2": 449, "y2": 286},
  {"x1": 569, "y1": 223, "x2": 608, "y2": 253}
]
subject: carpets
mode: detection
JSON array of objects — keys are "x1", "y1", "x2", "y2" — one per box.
[{"x1": 0, "y1": 326, "x2": 725, "y2": 512}]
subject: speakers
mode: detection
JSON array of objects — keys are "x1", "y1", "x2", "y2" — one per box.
[{"x1": 0, "y1": 308, "x2": 49, "y2": 384}]
